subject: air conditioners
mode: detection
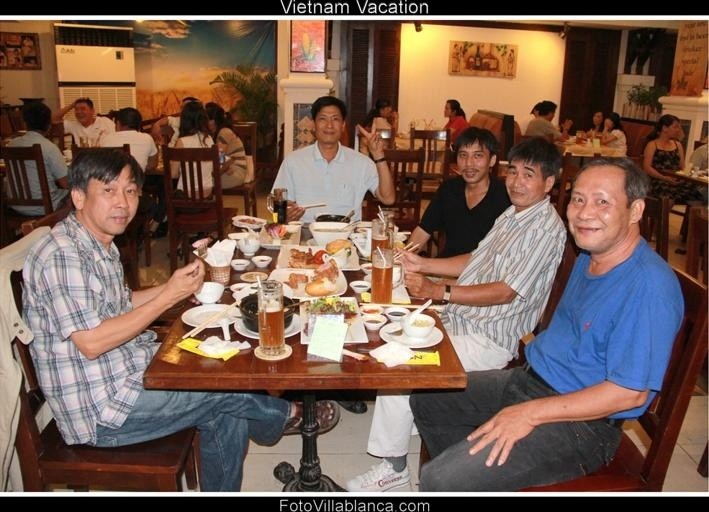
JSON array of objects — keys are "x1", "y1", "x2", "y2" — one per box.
[{"x1": 51, "y1": 23, "x2": 136, "y2": 126}]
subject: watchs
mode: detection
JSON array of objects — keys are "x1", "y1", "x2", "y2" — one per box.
[{"x1": 442, "y1": 284, "x2": 451, "y2": 304}]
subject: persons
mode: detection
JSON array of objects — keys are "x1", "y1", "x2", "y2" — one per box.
[
  {"x1": 360, "y1": 97, "x2": 399, "y2": 153},
  {"x1": 348, "y1": 137, "x2": 567, "y2": 493},
  {"x1": 408, "y1": 126, "x2": 512, "y2": 258},
  {"x1": 443, "y1": 100, "x2": 470, "y2": 143},
  {"x1": 271, "y1": 96, "x2": 396, "y2": 414},
  {"x1": 409, "y1": 156, "x2": 686, "y2": 492},
  {"x1": 19, "y1": 147, "x2": 341, "y2": 492},
  {"x1": 513, "y1": 100, "x2": 709, "y2": 242}
]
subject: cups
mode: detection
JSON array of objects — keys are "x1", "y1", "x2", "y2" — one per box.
[
  {"x1": 574, "y1": 129, "x2": 603, "y2": 147},
  {"x1": 60, "y1": 149, "x2": 74, "y2": 163},
  {"x1": 265, "y1": 187, "x2": 289, "y2": 224}
]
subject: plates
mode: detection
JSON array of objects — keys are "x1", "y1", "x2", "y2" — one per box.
[{"x1": 180, "y1": 210, "x2": 445, "y2": 362}]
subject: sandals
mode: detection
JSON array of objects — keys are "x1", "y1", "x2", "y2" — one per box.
[{"x1": 281, "y1": 398, "x2": 342, "y2": 435}]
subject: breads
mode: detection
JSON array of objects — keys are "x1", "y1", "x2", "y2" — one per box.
[{"x1": 305, "y1": 277, "x2": 336, "y2": 296}]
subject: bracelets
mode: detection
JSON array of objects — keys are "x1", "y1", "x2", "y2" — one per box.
[{"x1": 375, "y1": 158, "x2": 387, "y2": 163}]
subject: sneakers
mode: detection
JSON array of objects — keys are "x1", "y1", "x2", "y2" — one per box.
[
  {"x1": 336, "y1": 388, "x2": 368, "y2": 415},
  {"x1": 344, "y1": 458, "x2": 412, "y2": 492}
]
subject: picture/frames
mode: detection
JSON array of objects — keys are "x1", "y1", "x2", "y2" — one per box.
[{"x1": 288, "y1": 20, "x2": 328, "y2": 74}]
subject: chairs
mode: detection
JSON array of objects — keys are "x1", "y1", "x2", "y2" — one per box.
[{"x1": 0, "y1": 103, "x2": 708, "y2": 491}]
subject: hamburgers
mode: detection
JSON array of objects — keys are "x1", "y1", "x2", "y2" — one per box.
[{"x1": 326, "y1": 239, "x2": 352, "y2": 257}]
